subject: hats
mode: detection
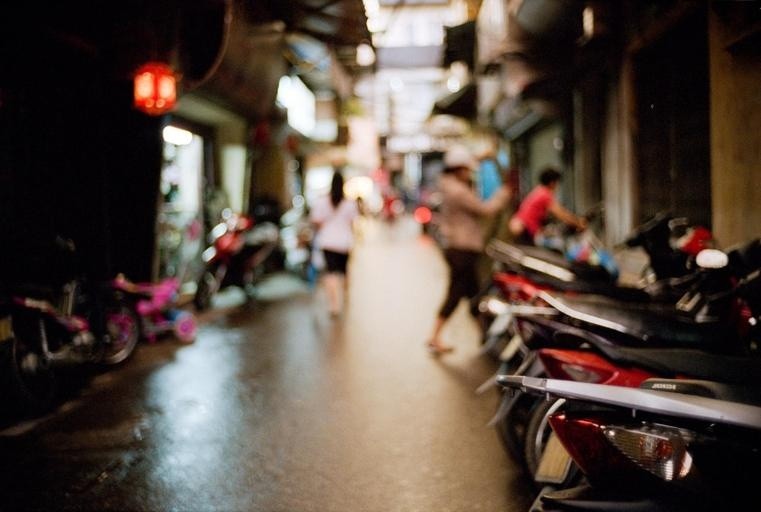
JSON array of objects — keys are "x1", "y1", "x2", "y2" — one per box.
[{"x1": 444, "y1": 146, "x2": 480, "y2": 171}]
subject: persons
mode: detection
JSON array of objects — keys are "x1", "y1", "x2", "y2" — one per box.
[
  {"x1": 310, "y1": 170, "x2": 361, "y2": 320},
  {"x1": 425, "y1": 138, "x2": 512, "y2": 358},
  {"x1": 508, "y1": 162, "x2": 587, "y2": 244}
]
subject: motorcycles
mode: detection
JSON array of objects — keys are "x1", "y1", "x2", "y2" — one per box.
[{"x1": 196, "y1": 206, "x2": 281, "y2": 308}]
